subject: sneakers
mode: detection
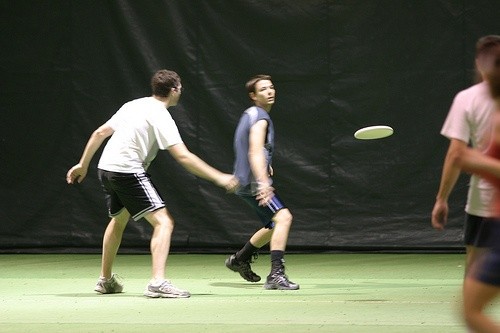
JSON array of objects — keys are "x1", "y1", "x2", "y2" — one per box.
[
  {"x1": 93, "y1": 274, "x2": 123, "y2": 294},
  {"x1": 145, "y1": 279, "x2": 191, "y2": 298},
  {"x1": 264, "y1": 273, "x2": 299, "y2": 290},
  {"x1": 225, "y1": 253, "x2": 261, "y2": 282}
]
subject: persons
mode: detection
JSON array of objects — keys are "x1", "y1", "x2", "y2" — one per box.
[
  {"x1": 451, "y1": 52, "x2": 500, "y2": 332},
  {"x1": 225, "y1": 75, "x2": 300, "y2": 290},
  {"x1": 432, "y1": 35, "x2": 500, "y2": 332},
  {"x1": 66, "y1": 70, "x2": 239, "y2": 298}
]
